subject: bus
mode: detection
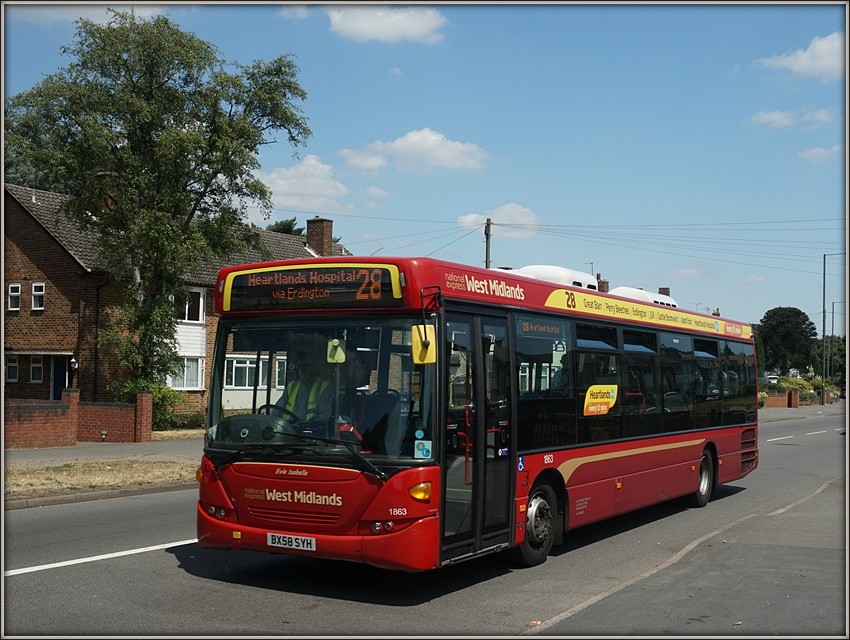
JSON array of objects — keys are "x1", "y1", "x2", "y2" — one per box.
[{"x1": 196, "y1": 255, "x2": 760, "y2": 573}]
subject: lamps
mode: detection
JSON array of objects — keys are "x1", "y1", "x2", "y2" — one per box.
[{"x1": 69, "y1": 356, "x2": 79, "y2": 371}]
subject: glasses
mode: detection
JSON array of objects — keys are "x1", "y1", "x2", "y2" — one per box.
[{"x1": 296, "y1": 364, "x2": 310, "y2": 370}]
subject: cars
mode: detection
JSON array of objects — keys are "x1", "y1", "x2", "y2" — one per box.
[{"x1": 766, "y1": 376, "x2": 779, "y2": 384}]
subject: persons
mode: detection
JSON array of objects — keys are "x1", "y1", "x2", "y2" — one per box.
[
  {"x1": 270, "y1": 359, "x2": 334, "y2": 425},
  {"x1": 550, "y1": 354, "x2": 570, "y2": 396}
]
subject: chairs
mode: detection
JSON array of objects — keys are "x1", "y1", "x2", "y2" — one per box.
[
  {"x1": 578, "y1": 389, "x2": 753, "y2": 442},
  {"x1": 334, "y1": 387, "x2": 402, "y2": 456}
]
